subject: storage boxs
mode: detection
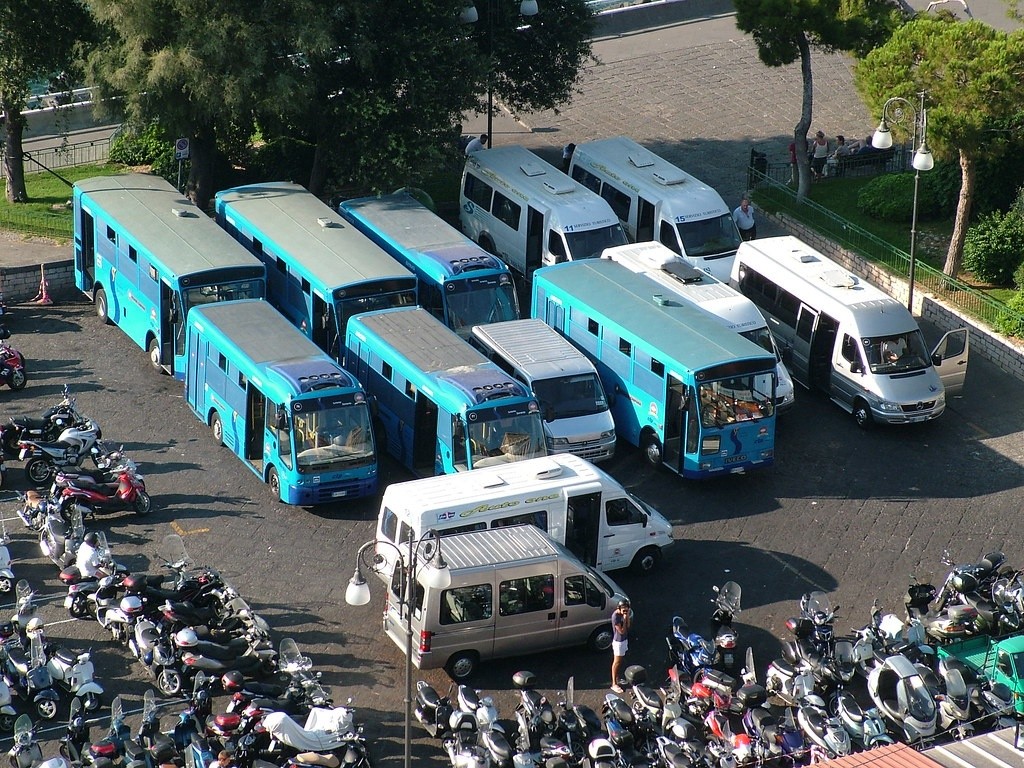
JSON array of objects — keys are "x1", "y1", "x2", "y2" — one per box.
[
  {"x1": 89, "y1": 740, "x2": 117, "y2": 759},
  {"x1": 736, "y1": 685, "x2": 768, "y2": 709},
  {"x1": 149, "y1": 743, "x2": 175, "y2": 765},
  {"x1": 624, "y1": 664, "x2": 647, "y2": 686},
  {"x1": 785, "y1": 618, "x2": 817, "y2": 638},
  {"x1": 512, "y1": 671, "x2": 536, "y2": 690},
  {"x1": 214, "y1": 713, "x2": 241, "y2": 731},
  {"x1": 947, "y1": 604, "x2": 978, "y2": 624},
  {"x1": 221, "y1": 670, "x2": 244, "y2": 694},
  {"x1": 173, "y1": 629, "x2": 199, "y2": 651},
  {"x1": 589, "y1": 738, "x2": 616, "y2": 761},
  {"x1": 120, "y1": 596, "x2": 143, "y2": 616},
  {"x1": 672, "y1": 723, "x2": 697, "y2": 742},
  {"x1": 953, "y1": 573, "x2": 977, "y2": 594},
  {"x1": 59, "y1": 566, "x2": 82, "y2": 585},
  {"x1": 123, "y1": 573, "x2": 147, "y2": 593}
]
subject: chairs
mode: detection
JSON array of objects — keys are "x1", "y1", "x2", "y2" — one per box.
[
  {"x1": 492, "y1": 417, "x2": 517, "y2": 444},
  {"x1": 446, "y1": 591, "x2": 471, "y2": 623}
]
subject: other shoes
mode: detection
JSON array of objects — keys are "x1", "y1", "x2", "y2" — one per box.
[
  {"x1": 620, "y1": 679, "x2": 628, "y2": 685},
  {"x1": 611, "y1": 686, "x2": 624, "y2": 693}
]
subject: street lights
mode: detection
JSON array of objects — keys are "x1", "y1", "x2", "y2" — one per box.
[
  {"x1": 461, "y1": 0, "x2": 540, "y2": 150},
  {"x1": 872, "y1": 95, "x2": 935, "y2": 315},
  {"x1": 345, "y1": 529, "x2": 450, "y2": 768}
]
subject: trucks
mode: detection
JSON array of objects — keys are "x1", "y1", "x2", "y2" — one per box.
[{"x1": 936, "y1": 632, "x2": 1024, "y2": 718}]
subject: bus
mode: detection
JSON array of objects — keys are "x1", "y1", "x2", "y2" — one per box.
[
  {"x1": 569, "y1": 137, "x2": 744, "y2": 284},
  {"x1": 601, "y1": 240, "x2": 794, "y2": 413},
  {"x1": 530, "y1": 256, "x2": 778, "y2": 483},
  {"x1": 74, "y1": 174, "x2": 269, "y2": 381},
  {"x1": 181, "y1": 297, "x2": 380, "y2": 504},
  {"x1": 345, "y1": 304, "x2": 543, "y2": 480},
  {"x1": 212, "y1": 180, "x2": 419, "y2": 367},
  {"x1": 338, "y1": 188, "x2": 523, "y2": 342},
  {"x1": 457, "y1": 141, "x2": 628, "y2": 286},
  {"x1": 733, "y1": 234, "x2": 970, "y2": 429}
]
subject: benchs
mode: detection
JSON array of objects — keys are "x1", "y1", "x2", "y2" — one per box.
[{"x1": 836, "y1": 148, "x2": 897, "y2": 176}]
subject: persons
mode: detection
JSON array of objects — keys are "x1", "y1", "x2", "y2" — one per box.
[
  {"x1": 563, "y1": 143, "x2": 575, "y2": 166},
  {"x1": 733, "y1": 200, "x2": 756, "y2": 240},
  {"x1": 884, "y1": 338, "x2": 909, "y2": 364},
  {"x1": 789, "y1": 141, "x2": 809, "y2": 164},
  {"x1": 465, "y1": 134, "x2": 487, "y2": 157},
  {"x1": 455, "y1": 124, "x2": 468, "y2": 147},
  {"x1": 822, "y1": 135, "x2": 849, "y2": 176},
  {"x1": 809, "y1": 131, "x2": 829, "y2": 184},
  {"x1": 852, "y1": 136, "x2": 872, "y2": 155},
  {"x1": 610, "y1": 598, "x2": 630, "y2": 693}
]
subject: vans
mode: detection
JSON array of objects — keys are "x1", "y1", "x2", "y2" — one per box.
[
  {"x1": 372, "y1": 454, "x2": 676, "y2": 580},
  {"x1": 471, "y1": 318, "x2": 618, "y2": 464},
  {"x1": 383, "y1": 523, "x2": 635, "y2": 684}
]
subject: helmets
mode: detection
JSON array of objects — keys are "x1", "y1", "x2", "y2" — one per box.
[
  {"x1": 618, "y1": 599, "x2": 629, "y2": 610},
  {"x1": 84, "y1": 533, "x2": 98, "y2": 546}
]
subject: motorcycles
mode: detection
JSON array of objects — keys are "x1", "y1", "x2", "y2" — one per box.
[
  {"x1": 0, "y1": 383, "x2": 370, "y2": 768},
  {"x1": 0, "y1": 331, "x2": 26, "y2": 390},
  {"x1": 414, "y1": 546, "x2": 1024, "y2": 768}
]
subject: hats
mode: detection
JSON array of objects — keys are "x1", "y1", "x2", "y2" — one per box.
[{"x1": 816, "y1": 131, "x2": 824, "y2": 138}]
